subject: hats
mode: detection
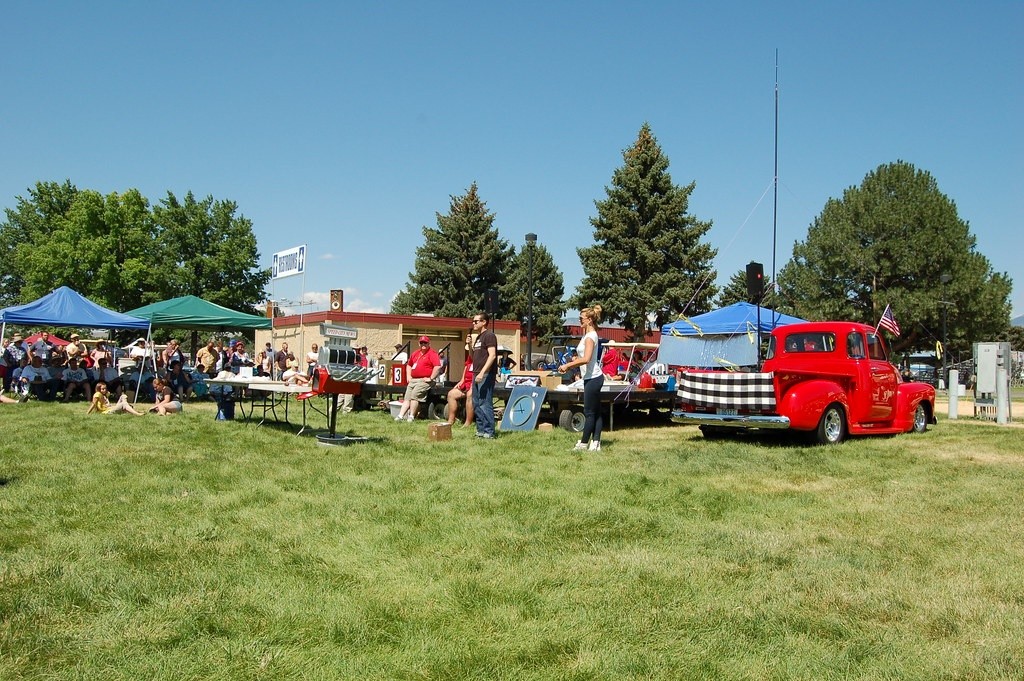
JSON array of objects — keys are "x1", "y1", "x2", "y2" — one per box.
[
  {"x1": 138, "y1": 337, "x2": 145, "y2": 341},
  {"x1": 13, "y1": 335, "x2": 24, "y2": 343},
  {"x1": 236, "y1": 341, "x2": 243, "y2": 347},
  {"x1": 361, "y1": 345, "x2": 366, "y2": 350},
  {"x1": 229, "y1": 339, "x2": 236, "y2": 347},
  {"x1": 394, "y1": 344, "x2": 402, "y2": 348},
  {"x1": 418, "y1": 336, "x2": 430, "y2": 343},
  {"x1": 94, "y1": 338, "x2": 106, "y2": 349},
  {"x1": 70, "y1": 333, "x2": 79, "y2": 339}
]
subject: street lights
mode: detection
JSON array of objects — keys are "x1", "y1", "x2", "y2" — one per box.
[{"x1": 524, "y1": 232, "x2": 538, "y2": 372}]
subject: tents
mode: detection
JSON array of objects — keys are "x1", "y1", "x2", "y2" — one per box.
[
  {"x1": 120, "y1": 294, "x2": 276, "y2": 405},
  {"x1": 0, "y1": 286, "x2": 160, "y2": 403}
]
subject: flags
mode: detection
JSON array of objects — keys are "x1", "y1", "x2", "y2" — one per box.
[{"x1": 880, "y1": 307, "x2": 900, "y2": 338}]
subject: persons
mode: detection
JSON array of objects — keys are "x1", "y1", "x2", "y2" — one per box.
[
  {"x1": 274, "y1": 342, "x2": 295, "y2": 371},
  {"x1": 148, "y1": 378, "x2": 182, "y2": 417},
  {"x1": 440, "y1": 312, "x2": 497, "y2": 438},
  {"x1": 435, "y1": 349, "x2": 447, "y2": 382},
  {"x1": 361, "y1": 346, "x2": 384, "y2": 368},
  {"x1": 498, "y1": 351, "x2": 517, "y2": 377},
  {"x1": 520, "y1": 354, "x2": 525, "y2": 371},
  {"x1": 282, "y1": 360, "x2": 308, "y2": 386},
  {"x1": 0, "y1": 331, "x2": 276, "y2": 404},
  {"x1": 87, "y1": 382, "x2": 146, "y2": 417},
  {"x1": 306, "y1": 344, "x2": 320, "y2": 376},
  {"x1": 394, "y1": 336, "x2": 441, "y2": 423},
  {"x1": 600, "y1": 340, "x2": 619, "y2": 377},
  {"x1": 558, "y1": 305, "x2": 604, "y2": 452},
  {"x1": 391, "y1": 344, "x2": 408, "y2": 364},
  {"x1": 326, "y1": 394, "x2": 355, "y2": 415}
]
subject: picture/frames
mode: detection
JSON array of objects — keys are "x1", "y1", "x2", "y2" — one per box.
[{"x1": 504, "y1": 375, "x2": 540, "y2": 388}]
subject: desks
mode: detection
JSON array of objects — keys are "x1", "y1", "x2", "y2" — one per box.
[{"x1": 203, "y1": 378, "x2": 331, "y2": 438}]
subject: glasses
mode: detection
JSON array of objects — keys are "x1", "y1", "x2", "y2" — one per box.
[
  {"x1": 420, "y1": 342, "x2": 428, "y2": 344},
  {"x1": 579, "y1": 316, "x2": 588, "y2": 320},
  {"x1": 101, "y1": 362, "x2": 107, "y2": 365}
]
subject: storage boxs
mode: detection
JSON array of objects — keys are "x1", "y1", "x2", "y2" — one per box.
[
  {"x1": 428, "y1": 422, "x2": 452, "y2": 441},
  {"x1": 541, "y1": 376, "x2": 562, "y2": 390},
  {"x1": 536, "y1": 419, "x2": 552, "y2": 430},
  {"x1": 511, "y1": 371, "x2": 553, "y2": 384},
  {"x1": 600, "y1": 381, "x2": 631, "y2": 391},
  {"x1": 389, "y1": 401, "x2": 408, "y2": 419}
]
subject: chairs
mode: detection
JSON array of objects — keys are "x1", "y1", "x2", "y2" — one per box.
[{"x1": 9, "y1": 379, "x2": 197, "y2": 402}]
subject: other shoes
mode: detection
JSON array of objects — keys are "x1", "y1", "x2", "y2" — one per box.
[
  {"x1": 394, "y1": 415, "x2": 404, "y2": 421},
  {"x1": 587, "y1": 439, "x2": 601, "y2": 451},
  {"x1": 572, "y1": 439, "x2": 589, "y2": 450},
  {"x1": 475, "y1": 431, "x2": 495, "y2": 439},
  {"x1": 407, "y1": 414, "x2": 415, "y2": 422}
]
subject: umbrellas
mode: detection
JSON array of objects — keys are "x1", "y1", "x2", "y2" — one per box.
[{"x1": 497, "y1": 345, "x2": 513, "y2": 355}]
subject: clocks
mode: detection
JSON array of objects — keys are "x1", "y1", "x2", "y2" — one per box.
[{"x1": 510, "y1": 395, "x2": 536, "y2": 427}]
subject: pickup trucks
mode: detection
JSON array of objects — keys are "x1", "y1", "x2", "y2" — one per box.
[{"x1": 668, "y1": 322, "x2": 937, "y2": 447}]
